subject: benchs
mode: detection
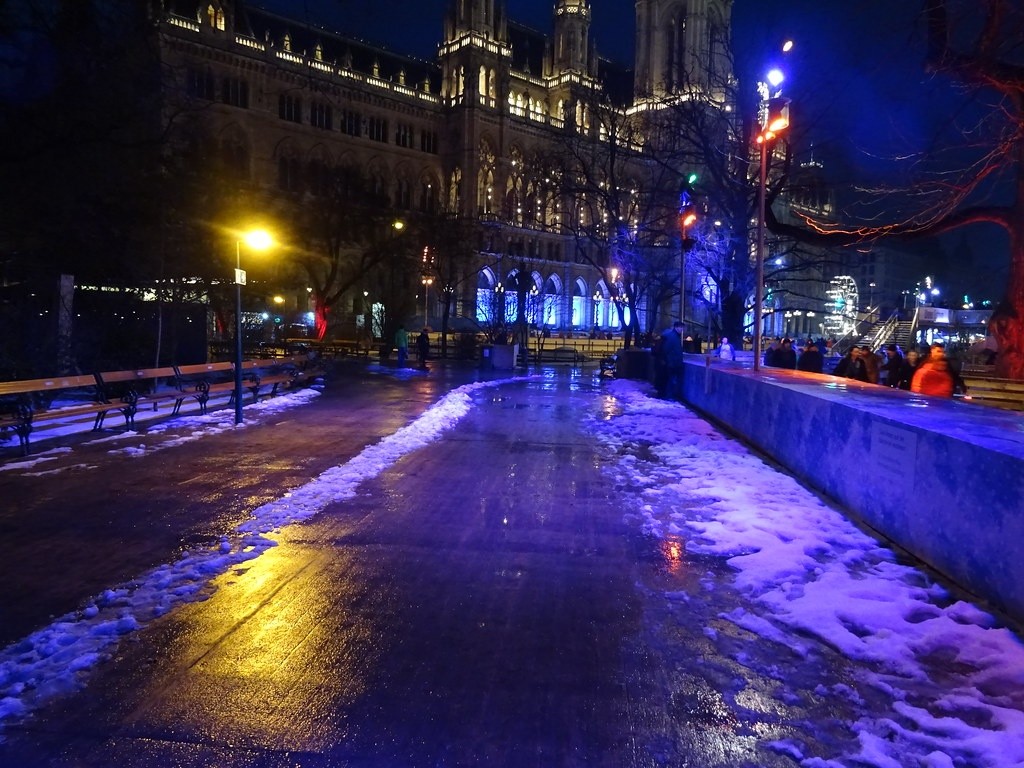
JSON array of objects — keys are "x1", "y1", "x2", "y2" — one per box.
[{"x1": 0, "y1": 356, "x2": 327, "y2": 456}]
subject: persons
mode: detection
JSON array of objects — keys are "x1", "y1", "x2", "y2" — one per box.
[
  {"x1": 395, "y1": 324, "x2": 408, "y2": 368},
  {"x1": 715, "y1": 336, "x2": 736, "y2": 361},
  {"x1": 530, "y1": 320, "x2": 614, "y2": 340},
  {"x1": 651, "y1": 321, "x2": 703, "y2": 400},
  {"x1": 743, "y1": 335, "x2": 968, "y2": 399},
  {"x1": 417, "y1": 328, "x2": 430, "y2": 364}
]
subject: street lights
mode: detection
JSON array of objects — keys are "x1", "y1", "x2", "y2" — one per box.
[
  {"x1": 679, "y1": 173, "x2": 700, "y2": 350},
  {"x1": 529, "y1": 285, "x2": 539, "y2": 330},
  {"x1": 902, "y1": 287, "x2": 910, "y2": 309},
  {"x1": 784, "y1": 310, "x2": 792, "y2": 338},
  {"x1": 793, "y1": 309, "x2": 802, "y2": 338},
  {"x1": 869, "y1": 282, "x2": 876, "y2": 311},
  {"x1": 231, "y1": 228, "x2": 273, "y2": 429},
  {"x1": 421, "y1": 278, "x2": 434, "y2": 332},
  {"x1": 753, "y1": 37, "x2": 794, "y2": 371},
  {"x1": 592, "y1": 291, "x2": 602, "y2": 332},
  {"x1": 807, "y1": 311, "x2": 815, "y2": 337}
]
